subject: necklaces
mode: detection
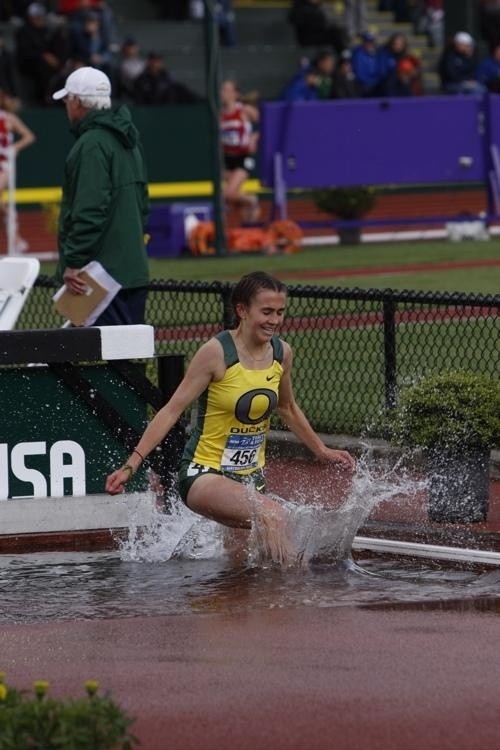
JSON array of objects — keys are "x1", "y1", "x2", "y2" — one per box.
[{"x1": 235, "y1": 328, "x2": 271, "y2": 362}]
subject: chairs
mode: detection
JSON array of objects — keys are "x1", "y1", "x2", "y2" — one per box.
[{"x1": 0, "y1": 253, "x2": 40, "y2": 332}]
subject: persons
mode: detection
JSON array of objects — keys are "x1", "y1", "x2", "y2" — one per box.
[
  {"x1": 51, "y1": 63, "x2": 154, "y2": 329},
  {"x1": 1, "y1": 1, "x2": 498, "y2": 220},
  {"x1": 103, "y1": 268, "x2": 356, "y2": 567}
]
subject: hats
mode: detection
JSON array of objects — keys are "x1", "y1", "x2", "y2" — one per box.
[
  {"x1": 455, "y1": 32, "x2": 475, "y2": 46},
  {"x1": 53, "y1": 65, "x2": 112, "y2": 98}
]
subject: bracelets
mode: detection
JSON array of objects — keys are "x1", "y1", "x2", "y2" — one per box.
[
  {"x1": 122, "y1": 463, "x2": 133, "y2": 479},
  {"x1": 133, "y1": 447, "x2": 145, "y2": 464}
]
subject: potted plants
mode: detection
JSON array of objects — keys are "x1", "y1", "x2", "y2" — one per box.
[
  {"x1": 387, "y1": 364, "x2": 500, "y2": 524},
  {"x1": 315, "y1": 182, "x2": 377, "y2": 249}
]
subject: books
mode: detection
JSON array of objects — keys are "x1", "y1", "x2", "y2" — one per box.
[
  {"x1": 53, "y1": 271, "x2": 110, "y2": 329},
  {"x1": 50, "y1": 261, "x2": 122, "y2": 328}
]
